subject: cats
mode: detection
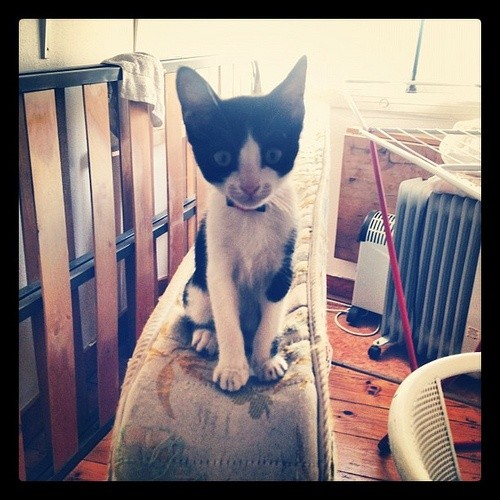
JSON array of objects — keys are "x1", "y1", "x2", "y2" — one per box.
[{"x1": 176, "y1": 55, "x2": 308, "y2": 392}]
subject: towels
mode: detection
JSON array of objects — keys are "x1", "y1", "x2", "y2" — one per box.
[{"x1": 100, "y1": 51, "x2": 166, "y2": 138}]
